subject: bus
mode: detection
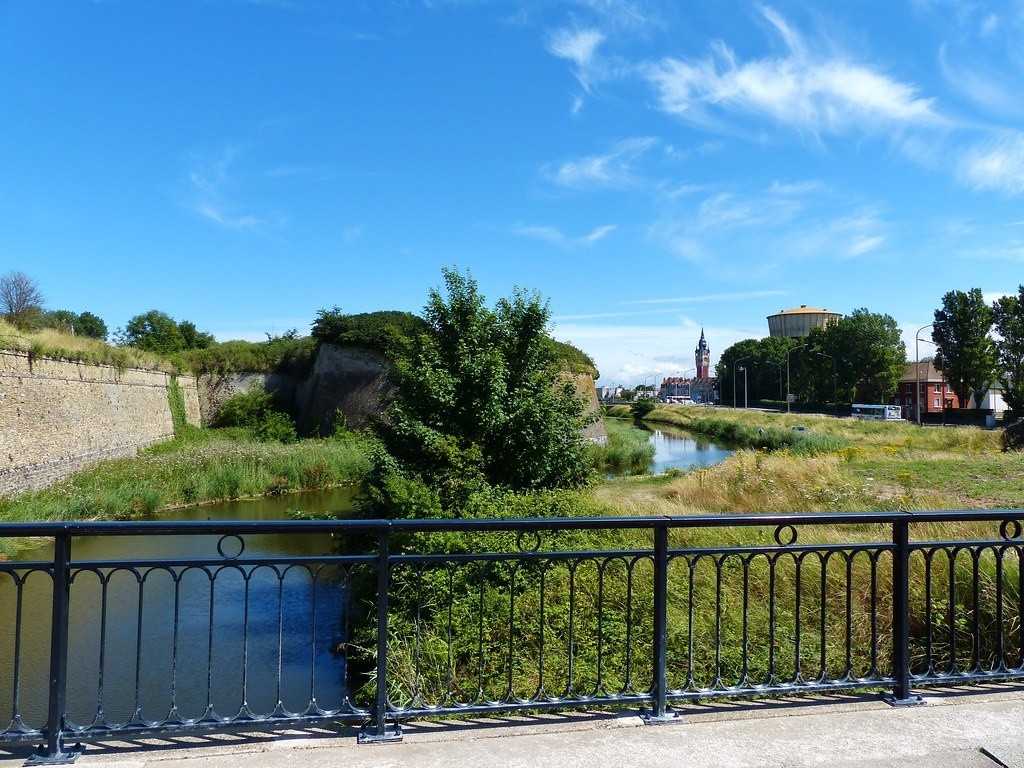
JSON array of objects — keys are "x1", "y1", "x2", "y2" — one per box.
[{"x1": 851, "y1": 403, "x2": 902, "y2": 420}]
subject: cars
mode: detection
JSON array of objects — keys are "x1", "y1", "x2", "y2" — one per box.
[{"x1": 653, "y1": 395, "x2": 697, "y2": 407}]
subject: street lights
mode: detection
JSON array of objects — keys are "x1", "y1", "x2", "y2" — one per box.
[
  {"x1": 683, "y1": 368, "x2": 696, "y2": 406},
  {"x1": 916, "y1": 320, "x2": 946, "y2": 425},
  {"x1": 653, "y1": 372, "x2": 664, "y2": 404},
  {"x1": 766, "y1": 360, "x2": 783, "y2": 412},
  {"x1": 643, "y1": 374, "x2": 652, "y2": 400},
  {"x1": 918, "y1": 338, "x2": 946, "y2": 426},
  {"x1": 816, "y1": 352, "x2": 837, "y2": 415},
  {"x1": 709, "y1": 370, "x2": 723, "y2": 404},
  {"x1": 666, "y1": 372, "x2": 680, "y2": 404},
  {"x1": 739, "y1": 366, "x2": 748, "y2": 408},
  {"x1": 733, "y1": 356, "x2": 750, "y2": 408},
  {"x1": 788, "y1": 343, "x2": 808, "y2": 413}
]
